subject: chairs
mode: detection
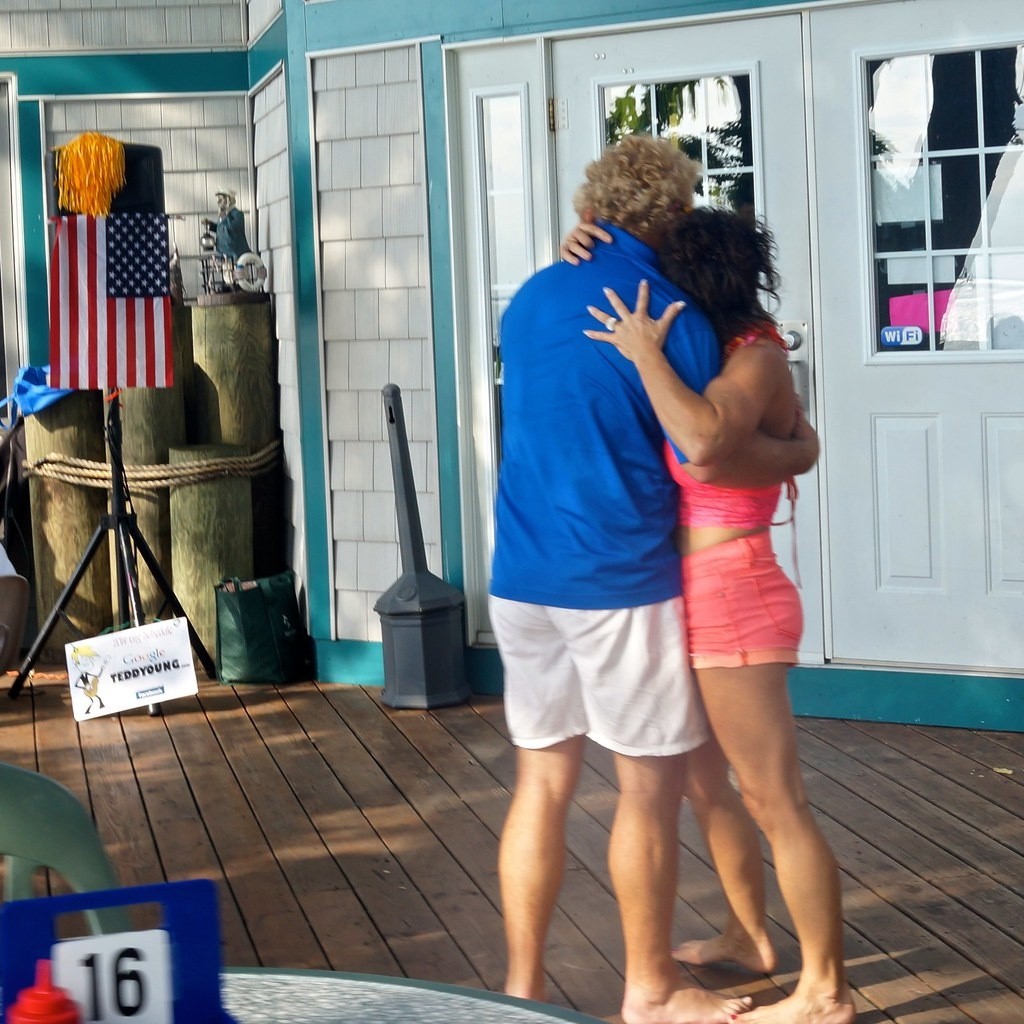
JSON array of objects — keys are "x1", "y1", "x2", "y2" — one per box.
[{"x1": 0, "y1": 762, "x2": 133, "y2": 937}]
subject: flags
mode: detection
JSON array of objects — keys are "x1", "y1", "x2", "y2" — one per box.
[{"x1": 47, "y1": 211, "x2": 174, "y2": 390}]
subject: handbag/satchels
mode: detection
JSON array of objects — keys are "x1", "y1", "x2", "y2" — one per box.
[
  {"x1": 0, "y1": 364, "x2": 76, "y2": 430},
  {"x1": 213, "y1": 571, "x2": 310, "y2": 685}
]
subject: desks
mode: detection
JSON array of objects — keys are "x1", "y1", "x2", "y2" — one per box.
[{"x1": 219, "y1": 966, "x2": 607, "y2": 1024}]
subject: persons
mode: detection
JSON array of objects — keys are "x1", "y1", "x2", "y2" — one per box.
[
  {"x1": 200, "y1": 184, "x2": 253, "y2": 291},
  {"x1": 496, "y1": 135, "x2": 857, "y2": 1024}
]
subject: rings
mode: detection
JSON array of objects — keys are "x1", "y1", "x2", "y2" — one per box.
[{"x1": 603, "y1": 315, "x2": 616, "y2": 332}]
